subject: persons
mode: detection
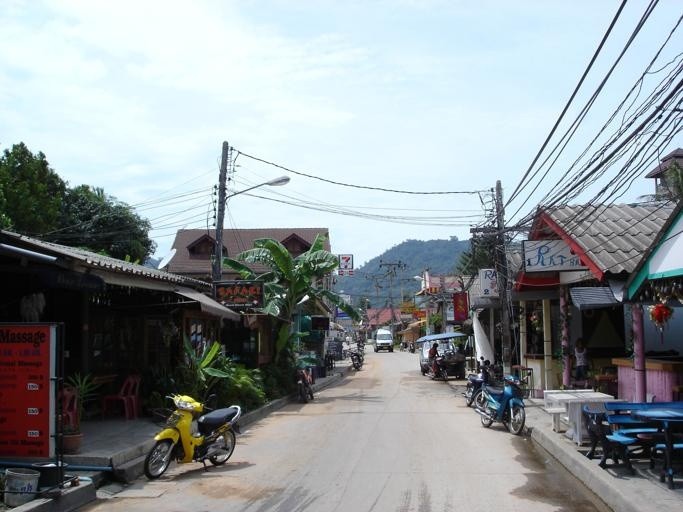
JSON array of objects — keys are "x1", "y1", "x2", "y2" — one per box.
[
  {"x1": 428, "y1": 342, "x2": 439, "y2": 365},
  {"x1": 573, "y1": 337, "x2": 588, "y2": 379}
]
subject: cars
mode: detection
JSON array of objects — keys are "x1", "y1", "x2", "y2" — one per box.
[{"x1": 418, "y1": 337, "x2": 455, "y2": 373}]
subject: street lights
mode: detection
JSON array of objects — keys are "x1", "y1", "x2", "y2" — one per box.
[{"x1": 210, "y1": 174, "x2": 291, "y2": 284}]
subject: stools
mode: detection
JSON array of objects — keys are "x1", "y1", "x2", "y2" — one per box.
[{"x1": 521, "y1": 368, "x2": 534, "y2": 398}]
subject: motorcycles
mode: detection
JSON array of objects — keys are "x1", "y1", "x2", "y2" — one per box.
[
  {"x1": 407, "y1": 340, "x2": 416, "y2": 354},
  {"x1": 460, "y1": 356, "x2": 503, "y2": 407},
  {"x1": 142, "y1": 392, "x2": 243, "y2": 481},
  {"x1": 422, "y1": 354, "x2": 449, "y2": 382},
  {"x1": 296, "y1": 367, "x2": 315, "y2": 403},
  {"x1": 350, "y1": 337, "x2": 366, "y2": 370},
  {"x1": 476, "y1": 369, "x2": 534, "y2": 435}
]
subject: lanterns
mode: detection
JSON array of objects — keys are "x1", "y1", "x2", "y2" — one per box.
[{"x1": 646, "y1": 304, "x2": 674, "y2": 345}]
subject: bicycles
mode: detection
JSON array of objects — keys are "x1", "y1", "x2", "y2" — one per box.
[{"x1": 399, "y1": 342, "x2": 405, "y2": 352}]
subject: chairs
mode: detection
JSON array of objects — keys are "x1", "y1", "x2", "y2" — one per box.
[
  {"x1": 54, "y1": 382, "x2": 83, "y2": 428},
  {"x1": 538, "y1": 389, "x2": 683, "y2": 491},
  {"x1": 101, "y1": 374, "x2": 143, "y2": 422}
]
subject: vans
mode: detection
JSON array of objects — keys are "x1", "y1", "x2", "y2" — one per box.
[{"x1": 372, "y1": 328, "x2": 393, "y2": 353}]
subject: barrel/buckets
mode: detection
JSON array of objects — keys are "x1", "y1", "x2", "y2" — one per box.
[
  {"x1": 1, "y1": 467, "x2": 41, "y2": 507},
  {"x1": 32, "y1": 462, "x2": 68, "y2": 487}
]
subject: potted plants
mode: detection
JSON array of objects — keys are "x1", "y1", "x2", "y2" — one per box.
[{"x1": 56, "y1": 369, "x2": 106, "y2": 455}]
